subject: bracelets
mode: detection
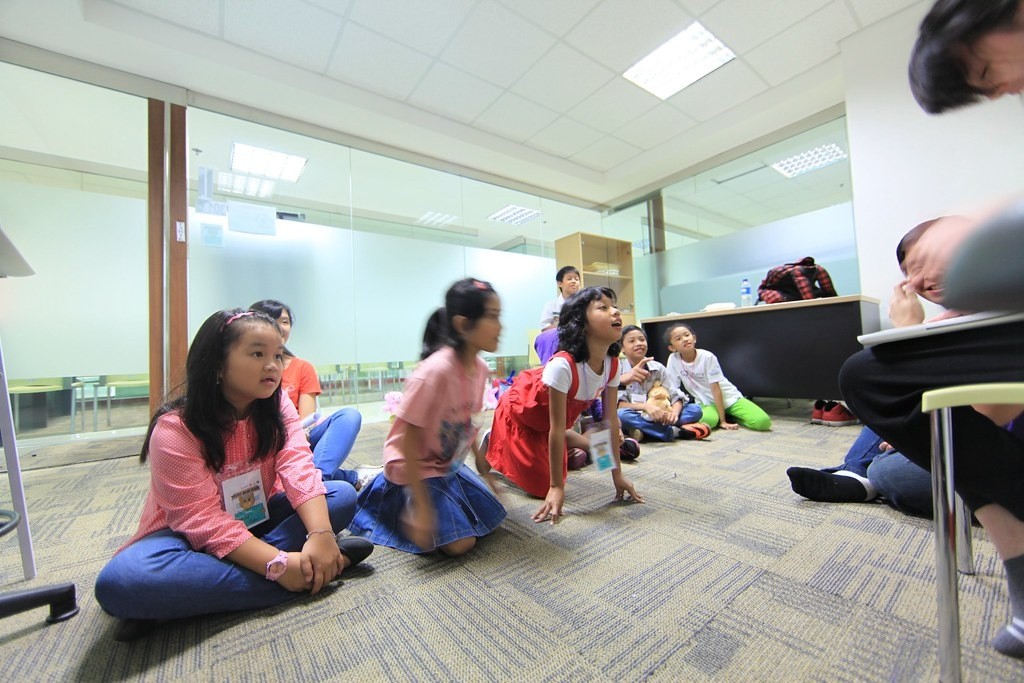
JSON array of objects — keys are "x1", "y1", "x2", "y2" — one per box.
[{"x1": 306, "y1": 530, "x2": 336, "y2": 539}]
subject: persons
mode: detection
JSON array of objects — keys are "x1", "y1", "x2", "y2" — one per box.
[
  {"x1": 475, "y1": 266, "x2": 772, "y2": 525},
  {"x1": 94, "y1": 308, "x2": 373, "y2": 642},
  {"x1": 249, "y1": 300, "x2": 385, "y2": 494},
  {"x1": 785, "y1": 0, "x2": 1024, "y2": 656},
  {"x1": 346, "y1": 276, "x2": 508, "y2": 557}
]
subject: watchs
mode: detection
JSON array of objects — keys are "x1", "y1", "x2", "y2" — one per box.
[{"x1": 265, "y1": 550, "x2": 288, "y2": 581}]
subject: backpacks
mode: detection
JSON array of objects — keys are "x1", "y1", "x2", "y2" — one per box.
[{"x1": 754, "y1": 256, "x2": 837, "y2": 305}]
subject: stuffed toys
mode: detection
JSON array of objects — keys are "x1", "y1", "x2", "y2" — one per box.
[
  {"x1": 384, "y1": 391, "x2": 404, "y2": 423},
  {"x1": 481, "y1": 379, "x2": 498, "y2": 412},
  {"x1": 642, "y1": 382, "x2": 674, "y2": 422}
]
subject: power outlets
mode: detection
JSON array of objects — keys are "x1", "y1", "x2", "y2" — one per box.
[{"x1": 176, "y1": 221, "x2": 186, "y2": 242}]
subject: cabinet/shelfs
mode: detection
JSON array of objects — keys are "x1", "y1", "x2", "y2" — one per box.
[{"x1": 555, "y1": 233, "x2": 636, "y2": 327}]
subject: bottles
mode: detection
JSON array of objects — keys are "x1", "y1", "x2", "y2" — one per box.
[{"x1": 741, "y1": 278, "x2": 752, "y2": 307}]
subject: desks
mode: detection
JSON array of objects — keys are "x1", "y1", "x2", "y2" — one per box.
[{"x1": 641, "y1": 295, "x2": 880, "y2": 408}]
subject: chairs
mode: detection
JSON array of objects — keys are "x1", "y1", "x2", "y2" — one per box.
[
  {"x1": 922, "y1": 382, "x2": 1024, "y2": 682},
  {"x1": 526, "y1": 330, "x2": 542, "y2": 368}
]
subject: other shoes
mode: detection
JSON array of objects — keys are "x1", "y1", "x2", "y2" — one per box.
[
  {"x1": 567, "y1": 447, "x2": 587, "y2": 471},
  {"x1": 676, "y1": 422, "x2": 712, "y2": 440},
  {"x1": 619, "y1": 438, "x2": 641, "y2": 461},
  {"x1": 629, "y1": 427, "x2": 643, "y2": 442},
  {"x1": 811, "y1": 399, "x2": 861, "y2": 425},
  {"x1": 353, "y1": 464, "x2": 386, "y2": 491},
  {"x1": 474, "y1": 427, "x2": 492, "y2": 475},
  {"x1": 335, "y1": 536, "x2": 375, "y2": 573}
]
